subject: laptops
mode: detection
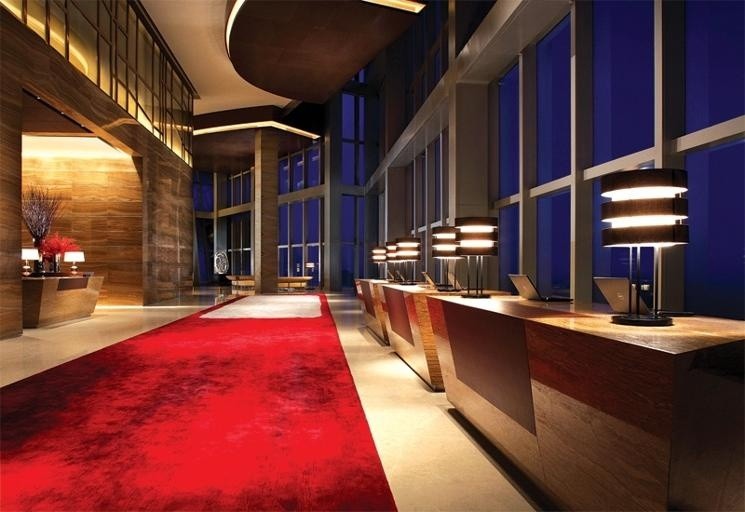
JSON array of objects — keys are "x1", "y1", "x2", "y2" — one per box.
[
  {"x1": 508, "y1": 274, "x2": 573, "y2": 301},
  {"x1": 421, "y1": 272, "x2": 453, "y2": 287},
  {"x1": 388, "y1": 271, "x2": 402, "y2": 280},
  {"x1": 445, "y1": 272, "x2": 484, "y2": 290},
  {"x1": 593, "y1": 277, "x2": 695, "y2": 317},
  {"x1": 396, "y1": 270, "x2": 420, "y2": 282}
]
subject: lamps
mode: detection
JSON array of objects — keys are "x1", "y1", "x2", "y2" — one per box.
[
  {"x1": 432, "y1": 226, "x2": 461, "y2": 292},
  {"x1": 64, "y1": 251, "x2": 86, "y2": 276},
  {"x1": 21, "y1": 247, "x2": 40, "y2": 276},
  {"x1": 602, "y1": 169, "x2": 690, "y2": 326},
  {"x1": 455, "y1": 218, "x2": 499, "y2": 298},
  {"x1": 370, "y1": 238, "x2": 422, "y2": 286}
]
